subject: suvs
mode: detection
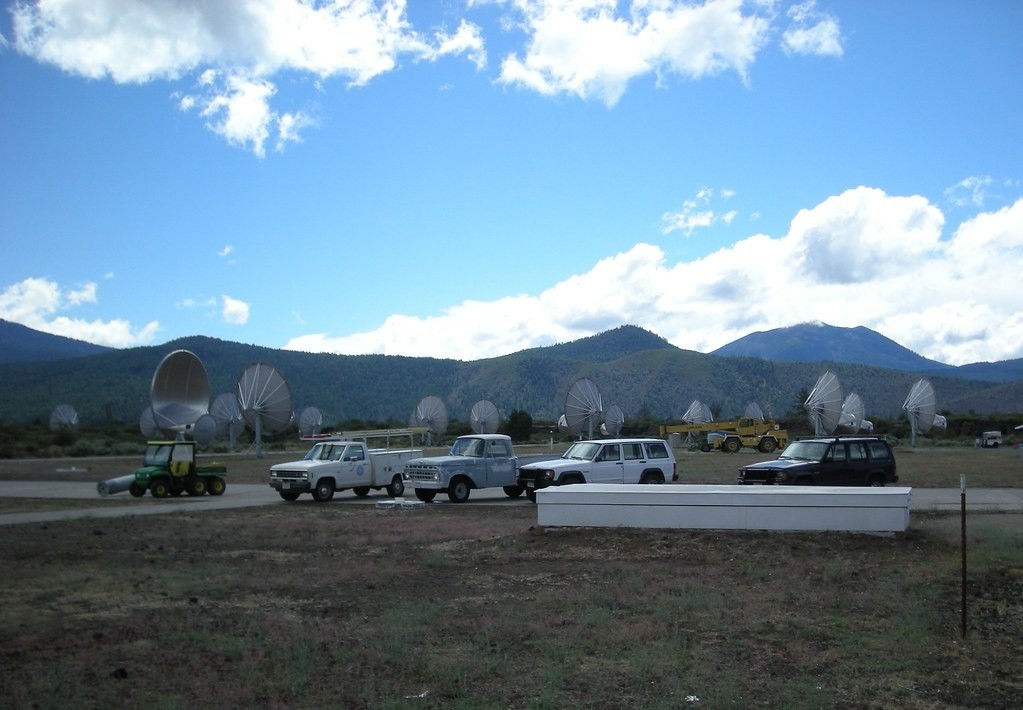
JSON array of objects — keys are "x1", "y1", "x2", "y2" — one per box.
[
  {"x1": 976, "y1": 431, "x2": 1002, "y2": 448},
  {"x1": 517, "y1": 438, "x2": 679, "y2": 503},
  {"x1": 735, "y1": 436, "x2": 899, "y2": 488}
]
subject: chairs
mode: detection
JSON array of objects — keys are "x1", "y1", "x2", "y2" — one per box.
[{"x1": 835, "y1": 449, "x2": 845, "y2": 460}]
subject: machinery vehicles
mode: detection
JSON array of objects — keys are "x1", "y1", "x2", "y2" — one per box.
[
  {"x1": 659, "y1": 415, "x2": 789, "y2": 455},
  {"x1": 130, "y1": 439, "x2": 229, "y2": 499}
]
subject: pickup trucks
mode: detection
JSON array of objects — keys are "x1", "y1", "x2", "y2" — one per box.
[
  {"x1": 269, "y1": 441, "x2": 423, "y2": 503},
  {"x1": 401, "y1": 434, "x2": 565, "y2": 502}
]
